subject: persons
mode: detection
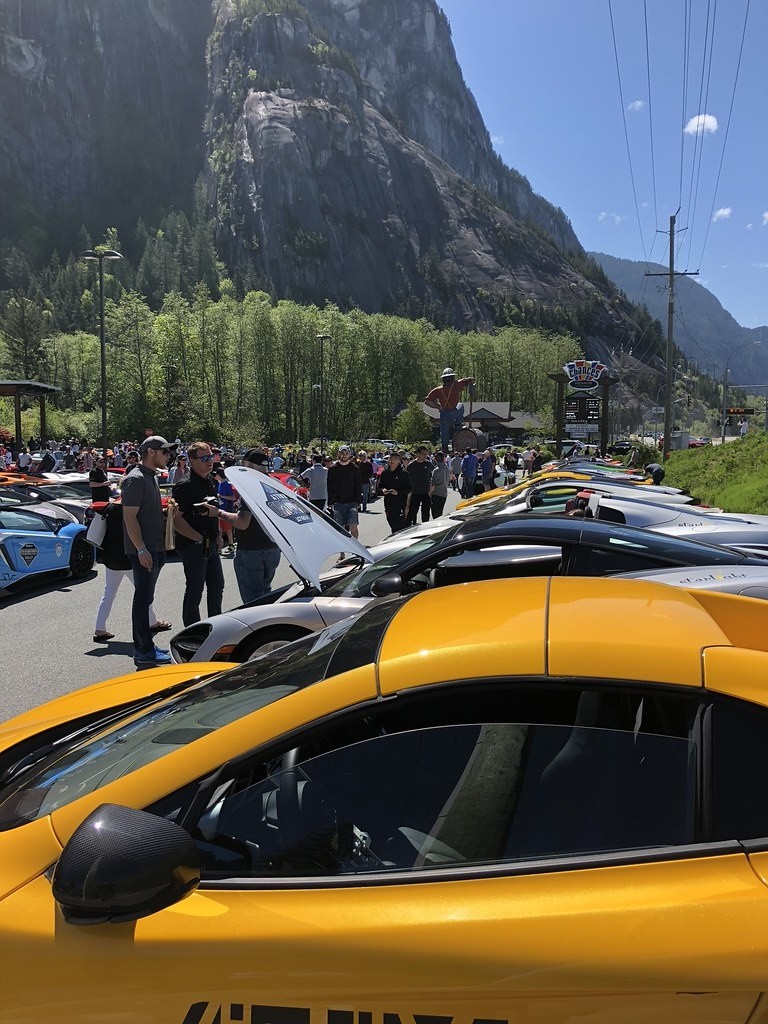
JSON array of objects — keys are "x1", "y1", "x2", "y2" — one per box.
[
  {"x1": 593, "y1": 448, "x2": 600, "y2": 458},
  {"x1": 573, "y1": 444, "x2": 582, "y2": 457},
  {"x1": 644, "y1": 463, "x2": 665, "y2": 485},
  {"x1": 737, "y1": 418, "x2": 748, "y2": 437},
  {"x1": 89, "y1": 456, "x2": 110, "y2": 503},
  {"x1": 425, "y1": 368, "x2": 476, "y2": 452},
  {"x1": 172, "y1": 441, "x2": 224, "y2": 626},
  {"x1": 207, "y1": 441, "x2": 236, "y2": 479},
  {"x1": 262, "y1": 444, "x2": 315, "y2": 473},
  {"x1": 585, "y1": 446, "x2": 590, "y2": 458},
  {"x1": 0, "y1": 436, "x2": 141, "y2": 475},
  {"x1": 202, "y1": 448, "x2": 281, "y2": 603},
  {"x1": 93, "y1": 498, "x2": 171, "y2": 642},
  {"x1": 167, "y1": 436, "x2": 190, "y2": 483},
  {"x1": 297, "y1": 445, "x2": 543, "y2": 538},
  {"x1": 627, "y1": 446, "x2": 637, "y2": 467},
  {"x1": 120, "y1": 435, "x2": 179, "y2": 663},
  {"x1": 216, "y1": 467, "x2": 237, "y2": 553}
]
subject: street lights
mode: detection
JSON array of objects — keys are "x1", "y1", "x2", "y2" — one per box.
[
  {"x1": 316, "y1": 332, "x2": 328, "y2": 456},
  {"x1": 721, "y1": 340, "x2": 762, "y2": 443},
  {"x1": 78, "y1": 248, "x2": 126, "y2": 476},
  {"x1": 673, "y1": 364, "x2": 682, "y2": 437},
  {"x1": 654, "y1": 382, "x2": 679, "y2": 448}
]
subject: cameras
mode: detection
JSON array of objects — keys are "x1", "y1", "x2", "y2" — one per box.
[{"x1": 192, "y1": 496, "x2": 219, "y2": 517}]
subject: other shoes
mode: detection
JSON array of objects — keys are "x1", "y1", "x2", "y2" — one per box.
[{"x1": 225, "y1": 545, "x2": 236, "y2": 553}]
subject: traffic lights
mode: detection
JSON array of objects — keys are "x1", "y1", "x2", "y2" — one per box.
[
  {"x1": 687, "y1": 395, "x2": 692, "y2": 407},
  {"x1": 729, "y1": 416, "x2": 734, "y2": 427},
  {"x1": 676, "y1": 426, "x2": 680, "y2": 431},
  {"x1": 717, "y1": 419, "x2": 721, "y2": 427}
]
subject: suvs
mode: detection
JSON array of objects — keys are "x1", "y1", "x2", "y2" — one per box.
[{"x1": 656, "y1": 435, "x2": 707, "y2": 452}]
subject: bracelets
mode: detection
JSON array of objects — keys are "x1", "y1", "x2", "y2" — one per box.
[{"x1": 138, "y1": 548, "x2": 146, "y2": 554}]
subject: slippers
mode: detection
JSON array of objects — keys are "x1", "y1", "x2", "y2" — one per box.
[
  {"x1": 149, "y1": 621, "x2": 171, "y2": 630},
  {"x1": 94, "y1": 632, "x2": 114, "y2": 640}
]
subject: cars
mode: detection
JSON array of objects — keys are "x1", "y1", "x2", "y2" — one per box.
[
  {"x1": 1, "y1": 575, "x2": 767, "y2": 1023},
  {"x1": 637, "y1": 431, "x2": 665, "y2": 438},
  {"x1": 169, "y1": 467, "x2": 768, "y2": 664},
  {"x1": 271, "y1": 471, "x2": 309, "y2": 500},
  {"x1": 333, "y1": 439, "x2": 768, "y2": 558},
  {"x1": 700, "y1": 437, "x2": 713, "y2": 446},
  {"x1": 0, "y1": 450, "x2": 175, "y2": 522},
  {"x1": 0, "y1": 505, "x2": 101, "y2": 588}
]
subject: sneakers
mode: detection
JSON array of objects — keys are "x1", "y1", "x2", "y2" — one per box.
[{"x1": 133, "y1": 645, "x2": 171, "y2": 663}]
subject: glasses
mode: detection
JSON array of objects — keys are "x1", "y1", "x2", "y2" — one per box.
[
  {"x1": 163, "y1": 450, "x2": 172, "y2": 456},
  {"x1": 194, "y1": 455, "x2": 214, "y2": 462}
]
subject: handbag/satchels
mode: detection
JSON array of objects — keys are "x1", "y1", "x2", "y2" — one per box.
[{"x1": 82, "y1": 503, "x2": 112, "y2": 550}]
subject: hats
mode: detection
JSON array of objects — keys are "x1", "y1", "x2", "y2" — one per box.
[
  {"x1": 129, "y1": 452, "x2": 138, "y2": 457},
  {"x1": 139, "y1": 436, "x2": 178, "y2": 451},
  {"x1": 244, "y1": 449, "x2": 270, "y2": 466},
  {"x1": 338, "y1": 445, "x2": 351, "y2": 452},
  {"x1": 359, "y1": 450, "x2": 367, "y2": 456},
  {"x1": 96, "y1": 456, "x2": 105, "y2": 462}
]
